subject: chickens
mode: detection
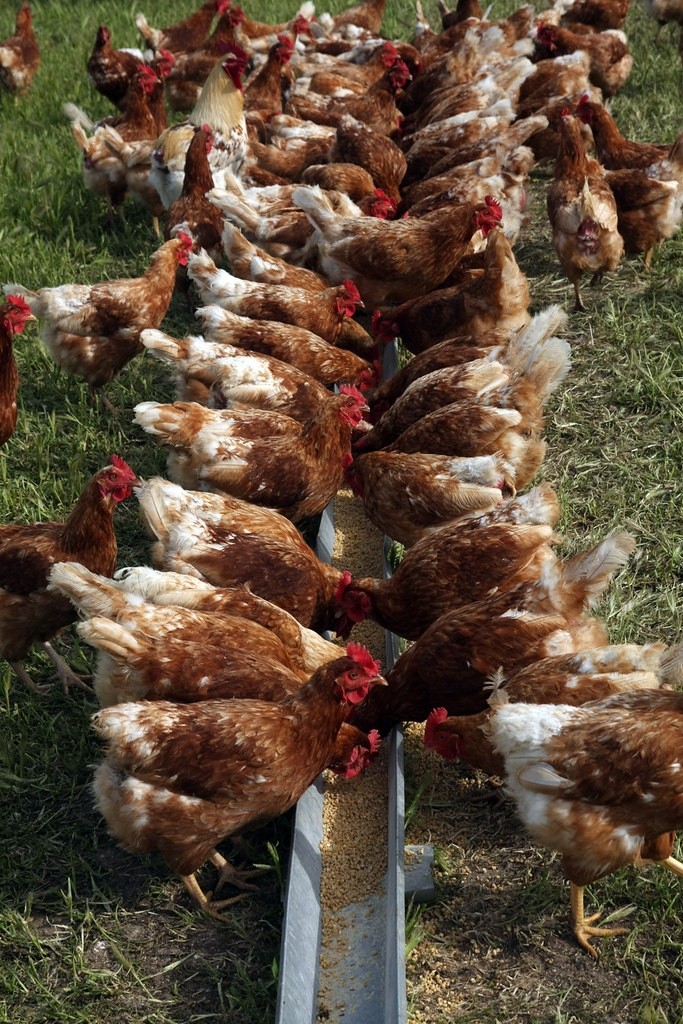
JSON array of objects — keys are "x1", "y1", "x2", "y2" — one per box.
[{"x1": 0, "y1": 0, "x2": 681, "y2": 963}]
